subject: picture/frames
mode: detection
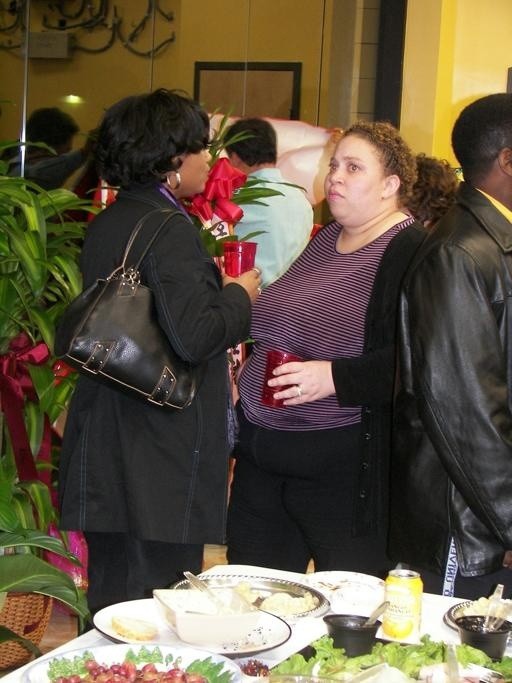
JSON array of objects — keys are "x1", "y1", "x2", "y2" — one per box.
[{"x1": 193, "y1": 61, "x2": 302, "y2": 126}]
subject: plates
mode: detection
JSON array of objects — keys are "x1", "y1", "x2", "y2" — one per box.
[
  {"x1": 443, "y1": 601, "x2": 512, "y2": 631},
  {"x1": 20, "y1": 644, "x2": 243, "y2": 683},
  {"x1": 92, "y1": 599, "x2": 291, "y2": 659},
  {"x1": 170, "y1": 573, "x2": 331, "y2": 624}
]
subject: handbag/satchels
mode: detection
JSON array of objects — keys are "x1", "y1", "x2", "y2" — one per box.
[{"x1": 53, "y1": 207, "x2": 201, "y2": 413}]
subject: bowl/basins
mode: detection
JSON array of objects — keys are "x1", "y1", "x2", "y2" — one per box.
[
  {"x1": 454, "y1": 615, "x2": 512, "y2": 664},
  {"x1": 323, "y1": 614, "x2": 382, "y2": 658}
]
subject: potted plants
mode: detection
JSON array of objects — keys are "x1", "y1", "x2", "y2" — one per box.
[{"x1": 1, "y1": 96, "x2": 309, "y2": 669}]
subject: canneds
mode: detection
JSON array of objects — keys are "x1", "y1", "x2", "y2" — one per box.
[{"x1": 380, "y1": 569, "x2": 424, "y2": 637}]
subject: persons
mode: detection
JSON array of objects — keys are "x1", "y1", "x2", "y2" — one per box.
[
  {"x1": 10, "y1": 108, "x2": 95, "y2": 193},
  {"x1": 387, "y1": 93, "x2": 512, "y2": 602},
  {"x1": 226, "y1": 119, "x2": 419, "y2": 581},
  {"x1": 58, "y1": 88, "x2": 261, "y2": 634},
  {"x1": 408, "y1": 154, "x2": 456, "y2": 225},
  {"x1": 224, "y1": 118, "x2": 312, "y2": 294}
]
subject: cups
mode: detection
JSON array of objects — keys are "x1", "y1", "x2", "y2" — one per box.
[
  {"x1": 222, "y1": 240, "x2": 257, "y2": 278},
  {"x1": 260, "y1": 349, "x2": 303, "y2": 410}
]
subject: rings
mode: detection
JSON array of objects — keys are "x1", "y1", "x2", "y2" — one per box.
[
  {"x1": 252, "y1": 268, "x2": 260, "y2": 273},
  {"x1": 297, "y1": 386, "x2": 303, "y2": 395},
  {"x1": 258, "y1": 288, "x2": 263, "y2": 297}
]
